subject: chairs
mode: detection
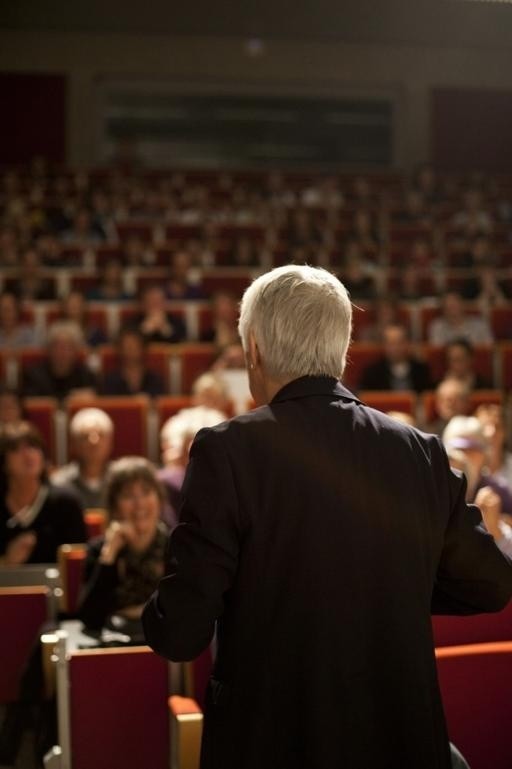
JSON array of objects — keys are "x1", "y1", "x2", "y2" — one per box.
[{"x1": 2, "y1": 154, "x2": 510, "y2": 763}]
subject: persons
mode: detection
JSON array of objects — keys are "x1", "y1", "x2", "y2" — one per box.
[{"x1": 1, "y1": 152, "x2": 511, "y2": 769}]
